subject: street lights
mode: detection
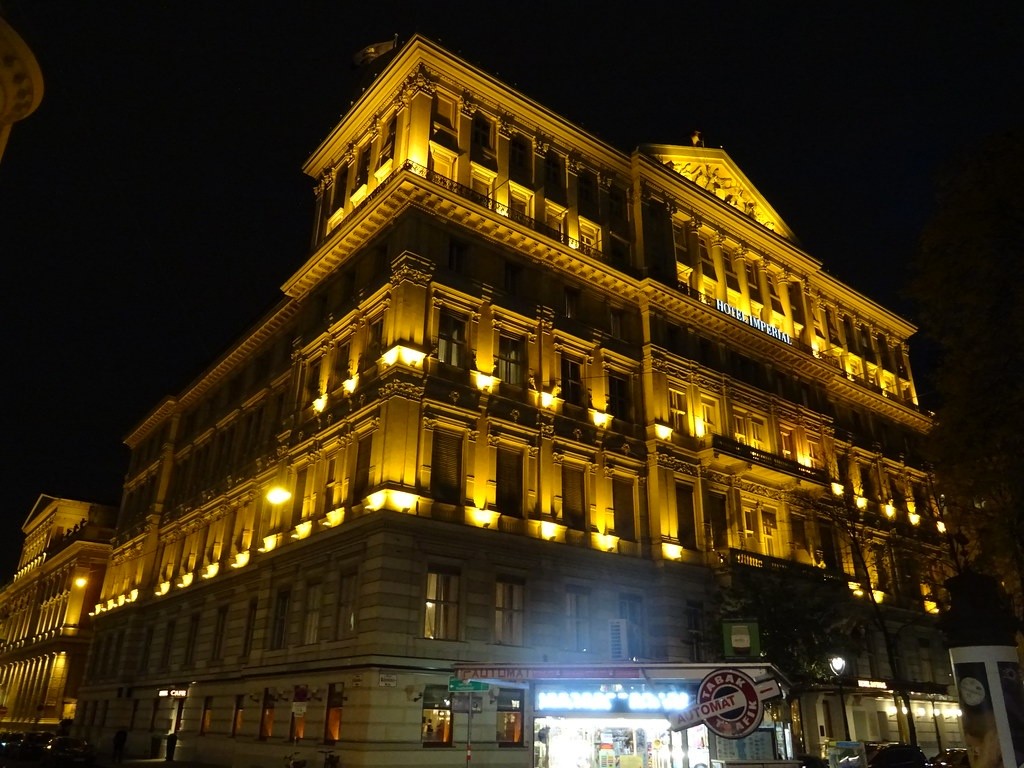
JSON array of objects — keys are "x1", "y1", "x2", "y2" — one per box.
[
  {"x1": 157, "y1": 688, "x2": 189, "y2": 734},
  {"x1": 828, "y1": 653, "x2": 857, "y2": 741}
]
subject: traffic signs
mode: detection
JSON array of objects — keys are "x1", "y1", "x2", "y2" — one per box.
[{"x1": 448, "y1": 679, "x2": 490, "y2": 691}]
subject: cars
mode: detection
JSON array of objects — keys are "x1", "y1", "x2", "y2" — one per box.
[
  {"x1": 0, "y1": 732, "x2": 26, "y2": 756},
  {"x1": 864, "y1": 741, "x2": 930, "y2": 768},
  {"x1": 39, "y1": 735, "x2": 98, "y2": 766},
  {"x1": 925, "y1": 747, "x2": 974, "y2": 768},
  {"x1": 17, "y1": 732, "x2": 56, "y2": 761},
  {"x1": 797, "y1": 753, "x2": 830, "y2": 768}
]
